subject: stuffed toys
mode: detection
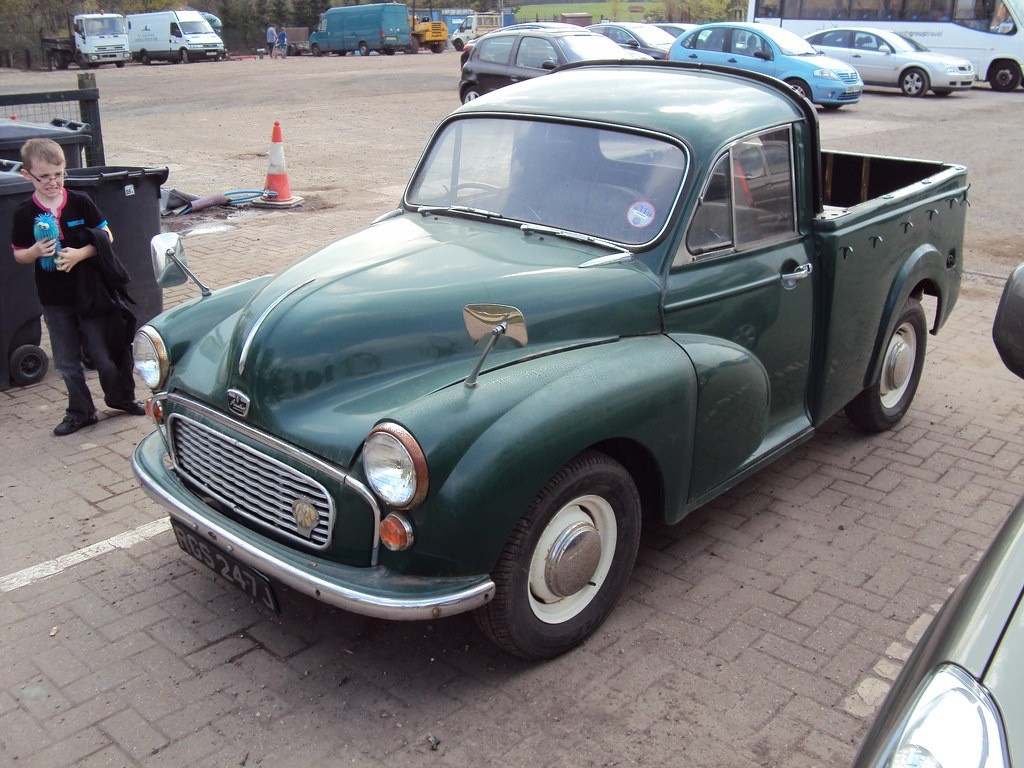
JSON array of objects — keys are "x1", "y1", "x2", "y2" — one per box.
[{"x1": 33, "y1": 213, "x2": 62, "y2": 272}]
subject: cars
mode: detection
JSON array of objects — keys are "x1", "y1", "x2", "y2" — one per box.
[
  {"x1": 455, "y1": 30, "x2": 634, "y2": 107},
  {"x1": 804, "y1": 25, "x2": 977, "y2": 97},
  {"x1": 649, "y1": 23, "x2": 712, "y2": 47},
  {"x1": 852, "y1": 262, "x2": 1024, "y2": 768},
  {"x1": 664, "y1": 22, "x2": 865, "y2": 109},
  {"x1": 583, "y1": 23, "x2": 678, "y2": 60},
  {"x1": 463, "y1": 23, "x2": 655, "y2": 63}
]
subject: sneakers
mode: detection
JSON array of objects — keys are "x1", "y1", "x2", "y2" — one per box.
[
  {"x1": 104, "y1": 399, "x2": 146, "y2": 415},
  {"x1": 54, "y1": 414, "x2": 98, "y2": 436}
]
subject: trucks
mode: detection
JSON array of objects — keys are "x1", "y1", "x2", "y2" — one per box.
[
  {"x1": 40, "y1": 8, "x2": 136, "y2": 68},
  {"x1": 280, "y1": 26, "x2": 314, "y2": 56},
  {"x1": 450, "y1": 11, "x2": 505, "y2": 50}
]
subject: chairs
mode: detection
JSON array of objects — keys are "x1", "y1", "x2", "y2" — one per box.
[
  {"x1": 634, "y1": 148, "x2": 763, "y2": 253},
  {"x1": 744, "y1": 35, "x2": 761, "y2": 57},
  {"x1": 793, "y1": 5, "x2": 953, "y2": 22}
]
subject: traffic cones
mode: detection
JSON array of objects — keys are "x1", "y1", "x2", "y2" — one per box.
[{"x1": 250, "y1": 120, "x2": 306, "y2": 209}]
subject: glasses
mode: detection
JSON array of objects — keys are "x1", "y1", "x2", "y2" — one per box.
[{"x1": 25, "y1": 168, "x2": 68, "y2": 184}]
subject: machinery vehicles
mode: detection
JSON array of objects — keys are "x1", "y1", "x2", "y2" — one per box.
[{"x1": 400, "y1": 12, "x2": 450, "y2": 55}]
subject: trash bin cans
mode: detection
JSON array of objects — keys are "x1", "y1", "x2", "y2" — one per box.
[
  {"x1": 62, "y1": 163, "x2": 171, "y2": 366},
  {"x1": 0, "y1": 156, "x2": 50, "y2": 390},
  {"x1": 0, "y1": 117, "x2": 94, "y2": 175}
]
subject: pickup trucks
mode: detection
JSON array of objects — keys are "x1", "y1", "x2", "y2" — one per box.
[{"x1": 127, "y1": 57, "x2": 972, "y2": 663}]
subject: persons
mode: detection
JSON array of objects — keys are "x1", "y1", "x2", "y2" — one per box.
[
  {"x1": 278, "y1": 26, "x2": 288, "y2": 59},
  {"x1": 9, "y1": 138, "x2": 146, "y2": 436},
  {"x1": 266, "y1": 23, "x2": 278, "y2": 58}
]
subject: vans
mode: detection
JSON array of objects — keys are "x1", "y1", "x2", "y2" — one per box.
[
  {"x1": 126, "y1": 11, "x2": 228, "y2": 64},
  {"x1": 310, "y1": 2, "x2": 412, "y2": 56}
]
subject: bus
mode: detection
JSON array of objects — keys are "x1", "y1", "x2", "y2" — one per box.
[{"x1": 748, "y1": 0, "x2": 1023, "y2": 92}]
